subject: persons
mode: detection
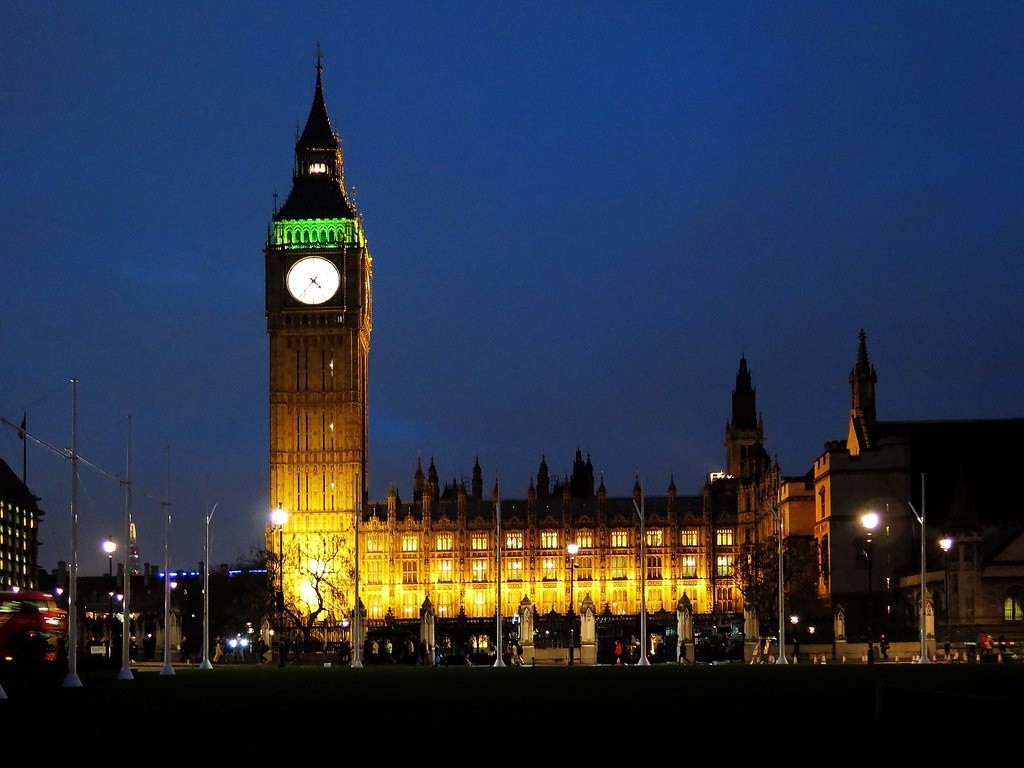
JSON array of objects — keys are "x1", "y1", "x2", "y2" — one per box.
[
  {"x1": 980, "y1": 631, "x2": 993, "y2": 654},
  {"x1": 362, "y1": 638, "x2": 397, "y2": 665},
  {"x1": 750, "y1": 635, "x2": 772, "y2": 664},
  {"x1": 612, "y1": 640, "x2": 625, "y2": 666},
  {"x1": 679, "y1": 641, "x2": 691, "y2": 665},
  {"x1": 790, "y1": 637, "x2": 800, "y2": 662},
  {"x1": 340, "y1": 642, "x2": 353, "y2": 666},
  {"x1": 213, "y1": 639, "x2": 224, "y2": 664},
  {"x1": 514, "y1": 641, "x2": 524, "y2": 665},
  {"x1": 258, "y1": 638, "x2": 268, "y2": 663},
  {"x1": 942, "y1": 636, "x2": 951, "y2": 659},
  {"x1": 463, "y1": 642, "x2": 473, "y2": 666},
  {"x1": 880, "y1": 635, "x2": 889, "y2": 660},
  {"x1": 283, "y1": 638, "x2": 293, "y2": 664},
  {"x1": 404, "y1": 639, "x2": 448, "y2": 667}
]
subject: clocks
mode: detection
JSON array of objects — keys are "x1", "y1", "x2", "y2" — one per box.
[{"x1": 286, "y1": 256, "x2": 341, "y2": 305}]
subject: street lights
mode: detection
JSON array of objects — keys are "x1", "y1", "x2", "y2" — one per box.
[
  {"x1": 269, "y1": 502, "x2": 289, "y2": 667},
  {"x1": 102, "y1": 534, "x2": 117, "y2": 668},
  {"x1": 860, "y1": 511, "x2": 880, "y2": 662},
  {"x1": 567, "y1": 543, "x2": 580, "y2": 665},
  {"x1": 939, "y1": 533, "x2": 953, "y2": 656}
]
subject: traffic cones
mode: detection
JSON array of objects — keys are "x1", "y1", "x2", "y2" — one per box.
[
  {"x1": 813, "y1": 653, "x2": 827, "y2": 665},
  {"x1": 792, "y1": 654, "x2": 799, "y2": 664},
  {"x1": 909, "y1": 651, "x2": 968, "y2": 663},
  {"x1": 862, "y1": 652, "x2": 868, "y2": 663},
  {"x1": 841, "y1": 654, "x2": 847, "y2": 664},
  {"x1": 975, "y1": 651, "x2": 981, "y2": 664},
  {"x1": 998, "y1": 650, "x2": 1003, "y2": 664}
]
